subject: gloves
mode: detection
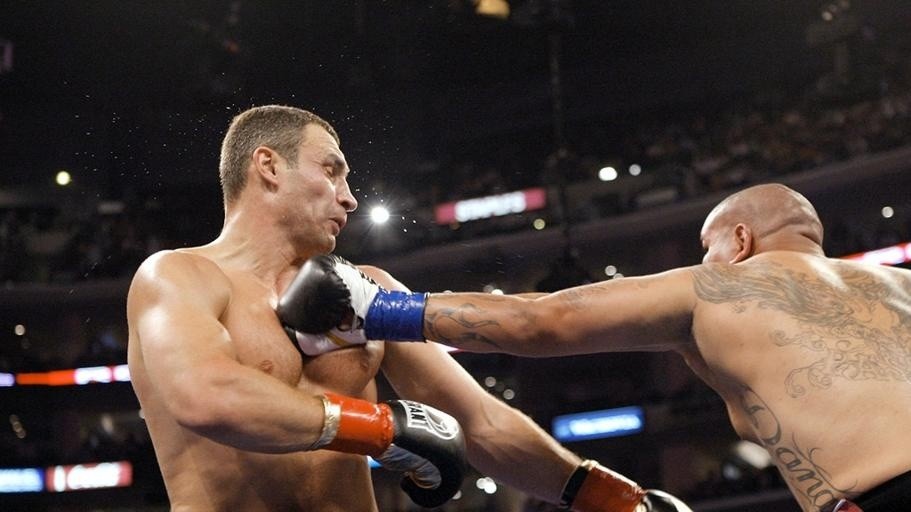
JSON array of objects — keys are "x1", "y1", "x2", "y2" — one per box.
[
  {"x1": 310, "y1": 392, "x2": 468, "y2": 508},
  {"x1": 558, "y1": 460, "x2": 694, "y2": 512},
  {"x1": 276, "y1": 254, "x2": 429, "y2": 356}
]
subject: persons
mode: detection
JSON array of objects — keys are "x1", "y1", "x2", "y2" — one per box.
[
  {"x1": 274, "y1": 181, "x2": 911, "y2": 512},
  {"x1": 122, "y1": 102, "x2": 695, "y2": 512}
]
